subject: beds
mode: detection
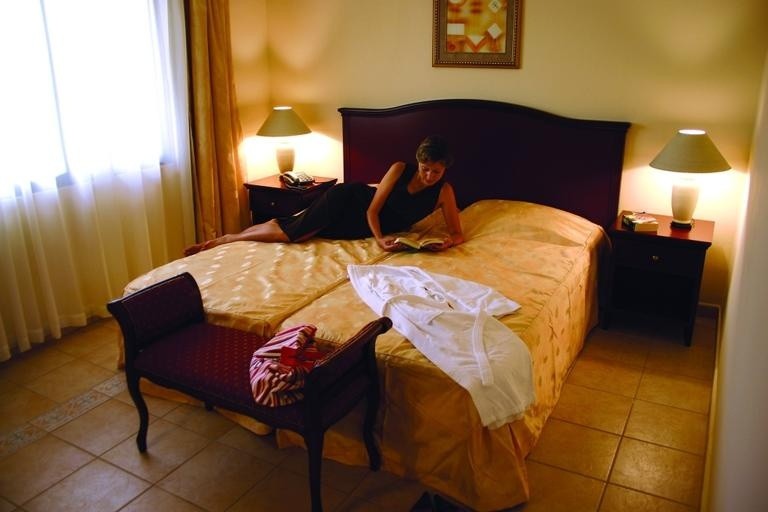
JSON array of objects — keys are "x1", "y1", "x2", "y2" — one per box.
[{"x1": 121, "y1": 99, "x2": 630, "y2": 512}]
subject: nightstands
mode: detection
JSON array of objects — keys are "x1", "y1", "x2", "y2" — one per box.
[
  {"x1": 599, "y1": 209, "x2": 715, "y2": 346},
  {"x1": 242, "y1": 175, "x2": 338, "y2": 227}
]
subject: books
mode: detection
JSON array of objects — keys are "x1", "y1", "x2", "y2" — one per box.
[
  {"x1": 622, "y1": 213, "x2": 659, "y2": 232},
  {"x1": 394, "y1": 231, "x2": 449, "y2": 250}
]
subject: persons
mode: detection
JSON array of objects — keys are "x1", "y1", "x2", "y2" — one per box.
[{"x1": 183, "y1": 136, "x2": 464, "y2": 257}]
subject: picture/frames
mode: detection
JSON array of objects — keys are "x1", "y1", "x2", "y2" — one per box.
[{"x1": 432, "y1": 0, "x2": 524, "y2": 70}]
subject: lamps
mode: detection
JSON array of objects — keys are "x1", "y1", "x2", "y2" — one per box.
[
  {"x1": 648, "y1": 129, "x2": 732, "y2": 229},
  {"x1": 255, "y1": 105, "x2": 311, "y2": 179}
]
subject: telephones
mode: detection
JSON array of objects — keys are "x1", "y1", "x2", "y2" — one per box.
[{"x1": 279, "y1": 171, "x2": 313, "y2": 185}]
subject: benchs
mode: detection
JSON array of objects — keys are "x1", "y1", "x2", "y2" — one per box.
[{"x1": 104, "y1": 272, "x2": 392, "y2": 512}]
